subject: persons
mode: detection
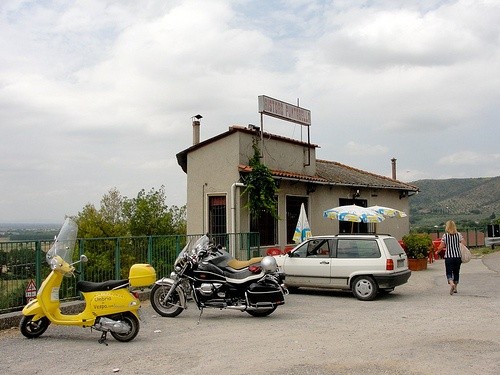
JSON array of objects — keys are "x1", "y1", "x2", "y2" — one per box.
[{"x1": 435, "y1": 221, "x2": 467, "y2": 296}]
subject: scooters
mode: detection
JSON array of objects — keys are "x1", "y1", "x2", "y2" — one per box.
[{"x1": 19, "y1": 217, "x2": 156, "y2": 346}]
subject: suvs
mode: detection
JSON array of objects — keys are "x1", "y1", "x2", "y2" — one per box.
[{"x1": 272, "y1": 232, "x2": 411, "y2": 300}]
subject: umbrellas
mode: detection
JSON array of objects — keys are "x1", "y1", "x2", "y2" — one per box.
[
  {"x1": 323, "y1": 204, "x2": 384, "y2": 234},
  {"x1": 293, "y1": 204, "x2": 312, "y2": 244},
  {"x1": 365, "y1": 205, "x2": 407, "y2": 233}
]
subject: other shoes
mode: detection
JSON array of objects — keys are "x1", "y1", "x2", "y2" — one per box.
[
  {"x1": 449, "y1": 284, "x2": 456, "y2": 295},
  {"x1": 454, "y1": 289, "x2": 457, "y2": 293}
]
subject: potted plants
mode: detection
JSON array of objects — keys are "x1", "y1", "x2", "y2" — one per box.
[{"x1": 402, "y1": 226, "x2": 433, "y2": 271}]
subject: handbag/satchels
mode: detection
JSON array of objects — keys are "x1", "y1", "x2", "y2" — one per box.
[{"x1": 459, "y1": 242, "x2": 472, "y2": 263}]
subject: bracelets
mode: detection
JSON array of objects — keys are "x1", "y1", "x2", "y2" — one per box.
[{"x1": 437, "y1": 250, "x2": 438, "y2": 254}]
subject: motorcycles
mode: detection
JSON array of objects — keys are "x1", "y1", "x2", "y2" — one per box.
[{"x1": 150, "y1": 233, "x2": 289, "y2": 318}]
